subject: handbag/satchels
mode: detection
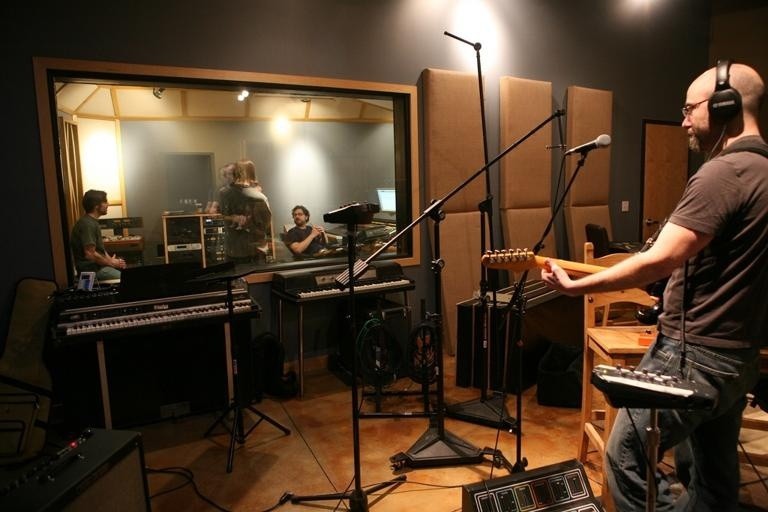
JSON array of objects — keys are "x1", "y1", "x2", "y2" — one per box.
[{"x1": 534, "y1": 340, "x2": 583, "y2": 408}]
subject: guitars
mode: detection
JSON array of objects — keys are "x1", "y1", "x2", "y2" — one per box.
[{"x1": 481, "y1": 238, "x2": 671, "y2": 299}]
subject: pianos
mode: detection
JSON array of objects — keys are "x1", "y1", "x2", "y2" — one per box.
[
  {"x1": 273, "y1": 259, "x2": 416, "y2": 302},
  {"x1": 56, "y1": 272, "x2": 262, "y2": 347}
]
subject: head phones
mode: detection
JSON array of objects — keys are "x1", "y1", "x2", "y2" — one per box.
[{"x1": 707, "y1": 61, "x2": 745, "y2": 138}]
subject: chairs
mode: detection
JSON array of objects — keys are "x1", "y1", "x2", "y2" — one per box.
[{"x1": 577, "y1": 242, "x2": 659, "y2": 512}]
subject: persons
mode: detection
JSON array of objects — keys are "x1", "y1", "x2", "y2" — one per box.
[
  {"x1": 541, "y1": 63, "x2": 768, "y2": 512},
  {"x1": 205, "y1": 161, "x2": 262, "y2": 228},
  {"x1": 218, "y1": 161, "x2": 270, "y2": 263},
  {"x1": 72, "y1": 190, "x2": 126, "y2": 280},
  {"x1": 284, "y1": 206, "x2": 329, "y2": 260}
]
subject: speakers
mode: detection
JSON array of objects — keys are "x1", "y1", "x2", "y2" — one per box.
[
  {"x1": 0, "y1": 426, "x2": 151, "y2": 512},
  {"x1": 162, "y1": 213, "x2": 207, "y2": 267}
]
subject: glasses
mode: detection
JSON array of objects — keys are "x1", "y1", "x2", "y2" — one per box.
[{"x1": 681, "y1": 98, "x2": 710, "y2": 118}]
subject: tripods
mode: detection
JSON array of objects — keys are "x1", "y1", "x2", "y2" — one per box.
[
  {"x1": 200, "y1": 290, "x2": 291, "y2": 474},
  {"x1": 290, "y1": 251, "x2": 408, "y2": 512}
]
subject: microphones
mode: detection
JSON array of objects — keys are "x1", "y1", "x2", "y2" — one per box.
[{"x1": 563, "y1": 134, "x2": 611, "y2": 155}]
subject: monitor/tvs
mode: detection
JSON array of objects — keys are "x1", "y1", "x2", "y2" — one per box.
[{"x1": 377, "y1": 186, "x2": 397, "y2": 213}]
